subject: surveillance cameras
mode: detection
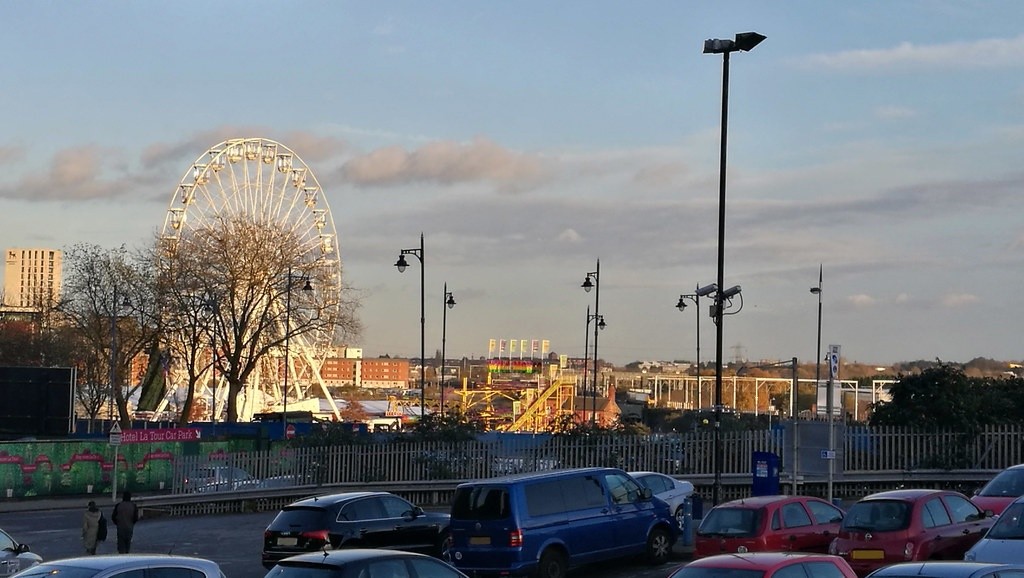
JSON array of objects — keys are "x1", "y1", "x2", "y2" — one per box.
[
  {"x1": 694, "y1": 283, "x2": 718, "y2": 296},
  {"x1": 723, "y1": 285, "x2": 741, "y2": 298}
]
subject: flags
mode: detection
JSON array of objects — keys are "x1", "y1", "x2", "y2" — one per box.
[{"x1": 490, "y1": 339, "x2": 567, "y2": 375}]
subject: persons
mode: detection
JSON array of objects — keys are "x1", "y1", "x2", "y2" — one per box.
[
  {"x1": 112, "y1": 492, "x2": 139, "y2": 554},
  {"x1": 81, "y1": 501, "x2": 102, "y2": 556}
]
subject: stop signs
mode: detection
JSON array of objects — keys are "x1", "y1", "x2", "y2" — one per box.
[
  {"x1": 285, "y1": 424, "x2": 296, "y2": 439},
  {"x1": 352, "y1": 424, "x2": 360, "y2": 437}
]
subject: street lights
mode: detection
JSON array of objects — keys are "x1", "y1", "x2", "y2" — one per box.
[
  {"x1": 205, "y1": 292, "x2": 217, "y2": 442},
  {"x1": 283, "y1": 264, "x2": 315, "y2": 448},
  {"x1": 675, "y1": 282, "x2": 700, "y2": 411},
  {"x1": 440, "y1": 281, "x2": 457, "y2": 418},
  {"x1": 392, "y1": 231, "x2": 425, "y2": 435},
  {"x1": 580, "y1": 259, "x2": 607, "y2": 429},
  {"x1": 110, "y1": 284, "x2": 132, "y2": 434},
  {"x1": 703, "y1": 32, "x2": 766, "y2": 505},
  {"x1": 810, "y1": 263, "x2": 822, "y2": 420}
]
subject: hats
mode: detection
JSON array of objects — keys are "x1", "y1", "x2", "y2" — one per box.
[{"x1": 86, "y1": 501, "x2": 96, "y2": 508}]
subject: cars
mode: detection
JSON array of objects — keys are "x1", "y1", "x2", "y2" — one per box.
[
  {"x1": 607, "y1": 472, "x2": 697, "y2": 530},
  {"x1": 0, "y1": 529, "x2": 44, "y2": 578},
  {"x1": 264, "y1": 550, "x2": 468, "y2": 578},
  {"x1": 183, "y1": 465, "x2": 314, "y2": 496},
  {"x1": 667, "y1": 463, "x2": 1024, "y2": 578},
  {"x1": 8, "y1": 554, "x2": 226, "y2": 578},
  {"x1": 262, "y1": 491, "x2": 451, "y2": 570},
  {"x1": 447, "y1": 468, "x2": 680, "y2": 578}
]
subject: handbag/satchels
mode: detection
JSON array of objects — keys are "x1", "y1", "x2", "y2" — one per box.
[{"x1": 97, "y1": 511, "x2": 108, "y2": 542}]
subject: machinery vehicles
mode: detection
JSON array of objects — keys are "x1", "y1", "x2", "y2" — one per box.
[{"x1": 374, "y1": 418, "x2": 403, "y2": 435}]
